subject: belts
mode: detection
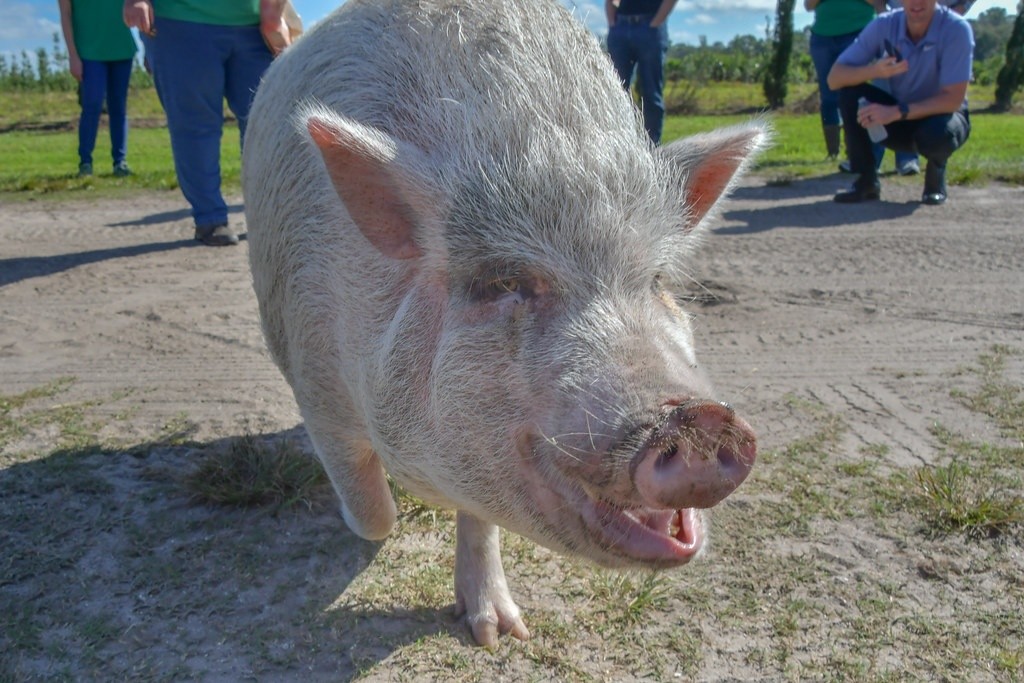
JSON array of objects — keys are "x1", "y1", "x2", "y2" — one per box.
[{"x1": 617, "y1": 15, "x2": 647, "y2": 22}]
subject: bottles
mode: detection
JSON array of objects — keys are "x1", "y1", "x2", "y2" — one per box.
[{"x1": 857, "y1": 96, "x2": 888, "y2": 143}]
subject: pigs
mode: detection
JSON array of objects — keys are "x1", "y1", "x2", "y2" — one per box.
[{"x1": 240, "y1": 0, "x2": 775, "y2": 652}]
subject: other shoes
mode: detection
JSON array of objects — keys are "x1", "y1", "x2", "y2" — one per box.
[
  {"x1": 114, "y1": 161, "x2": 136, "y2": 177},
  {"x1": 77, "y1": 163, "x2": 93, "y2": 177},
  {"x1": 990, "y1": 101, "x2": 1011, "y2": 113},
  {"x1": 922, "y1": 163, "x2": 947, "y2": 204},
  {"x1": 835, "y1": 180, "x2": 880, "y2": 202},
  {"x1": 896, "y1": 158, "x2": 921, "y2": 176},
  {"x1": 195, "y1": 222, "x2": 239, "y2": 246},
  {"x1": 839, "y1": 158, "x2": 851, "y2": 171}
]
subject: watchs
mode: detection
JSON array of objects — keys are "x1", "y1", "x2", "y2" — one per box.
[{"x1": 897, "y1": 103, "x2": 910, "y2": 120}]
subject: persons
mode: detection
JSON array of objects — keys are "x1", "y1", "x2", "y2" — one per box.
[
  {"x1": 605, "y1": 1, "x2": 679, "y2": 146},
  {"x1": 827, "y1": 0, "x2": 976, "y2": 205},
  {"x1": 838, "y1": 0, "x2": 978, "y2": 176},
  {"x1": 122, "y1": 0, "x2": 293, "y2": 246},
  {"x1": 59, "y1": 0, "x2": 138, "y2": 178},
  {"x1": 987, "y1": 0, "x2": 1024, "y2": 114},
  {"x1": 805, "y1": 0, "x2": 877, "y2": 162}
]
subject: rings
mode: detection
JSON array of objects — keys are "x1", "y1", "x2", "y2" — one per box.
[{"x1": 868, "y1": 117, "x2": 871, "y2": 121}]
